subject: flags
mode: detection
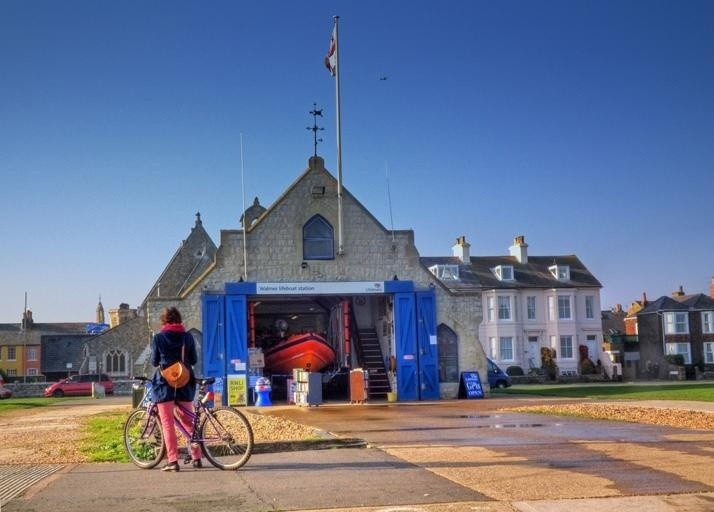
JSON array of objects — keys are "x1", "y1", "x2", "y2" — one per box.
[{"x1": 324, "y1": 26, "x2": 336, "y2": 76}]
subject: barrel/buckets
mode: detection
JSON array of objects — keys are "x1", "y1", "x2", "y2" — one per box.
[{"x1": 386, "y1": 392, "x2": 395, "y2": 402}]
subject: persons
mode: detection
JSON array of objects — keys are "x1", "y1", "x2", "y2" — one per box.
[{"x1": 151, "y1": 307, "x2": 202, "y2": 472}]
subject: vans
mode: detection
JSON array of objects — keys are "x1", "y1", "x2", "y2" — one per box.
[{"x1": 44, "y1": 374, "x2": 115, "y2": 397}]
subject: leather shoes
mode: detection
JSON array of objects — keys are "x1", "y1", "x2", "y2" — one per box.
[
  {"x1": 192, "y1": 459, "x2": 202, "y2": 468},
  {"x1": 160, "y1": 463, "x2": 179, "y2": 472}
]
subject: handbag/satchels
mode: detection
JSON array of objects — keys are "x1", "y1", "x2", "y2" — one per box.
[{"x1": 161, "y1": 361, "x2": 190, "y2": 388}]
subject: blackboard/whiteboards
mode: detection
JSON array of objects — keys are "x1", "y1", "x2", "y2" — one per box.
[{"x1": 461, "y1": 371, "x2": 484, "y2": 399}]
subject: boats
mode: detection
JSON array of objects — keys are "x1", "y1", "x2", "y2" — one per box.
[{"x1": 262, "y1": 333, "x2": 337, "y2": 374}]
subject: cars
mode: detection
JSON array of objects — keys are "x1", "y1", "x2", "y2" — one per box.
[{"x1": 486, "y1": 357, "x2": 512, "y2": 389}]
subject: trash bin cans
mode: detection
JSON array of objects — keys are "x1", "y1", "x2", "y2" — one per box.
[{"x1": 133, "y1": 388, "x2": 144, "y2": 408}]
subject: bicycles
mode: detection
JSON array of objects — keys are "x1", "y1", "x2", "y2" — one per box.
[{"x1": 124, "y1": 375, "x2": 254, "y2": 470}]
